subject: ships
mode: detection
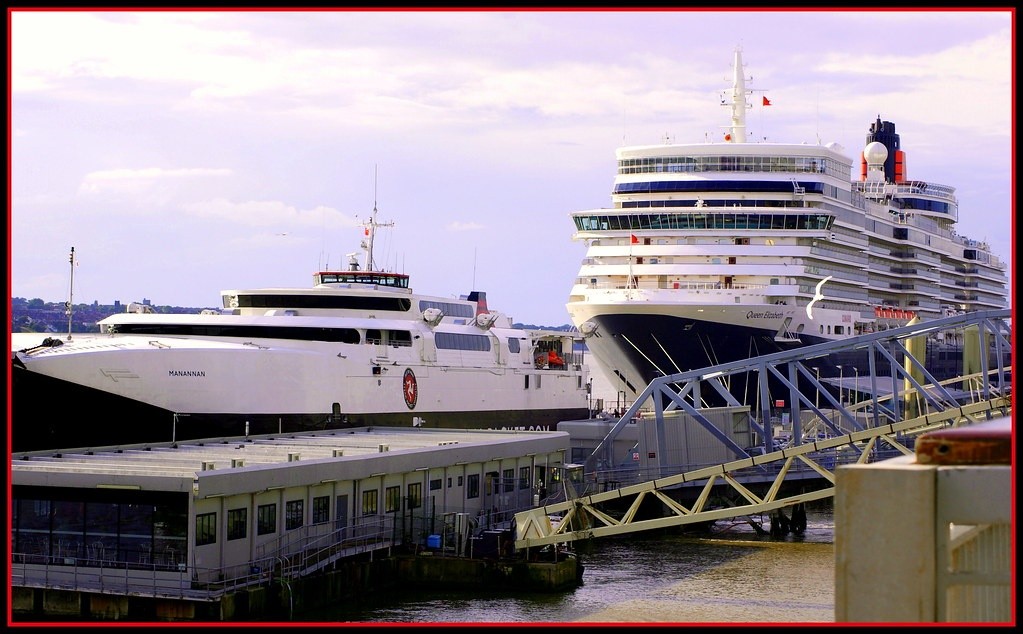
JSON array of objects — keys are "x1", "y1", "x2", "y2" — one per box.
[
  {"x1": 11, "y1": 182, "x2": 597, "y2": 456},
  {"x1": 560, "y1": 46, "x2": 1011, "y2": 434}
]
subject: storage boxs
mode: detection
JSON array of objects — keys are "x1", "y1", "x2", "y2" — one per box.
[{"x1": 427, "y1": 534, "x2": 442, "y2": 548}]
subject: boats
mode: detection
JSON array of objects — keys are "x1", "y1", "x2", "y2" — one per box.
[{"x1": 378, "y1": 542, "x2": 581, "y2": 596}]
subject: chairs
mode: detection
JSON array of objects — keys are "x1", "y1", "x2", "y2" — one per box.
[{"x1": 30, "y1": 538, "x2": 175, "y2": 570}]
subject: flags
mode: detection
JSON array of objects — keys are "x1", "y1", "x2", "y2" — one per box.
[
  {"x1": 632, "y1": 234, "x2": 639, "y2": 243},
  {"x1": 763, "y1": 96, "x2": 771, "y2": 105}
]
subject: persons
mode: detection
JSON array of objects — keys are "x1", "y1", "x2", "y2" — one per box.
[{"x1": 612, "y1": 409, "x2": 621, "y2": 418}]
